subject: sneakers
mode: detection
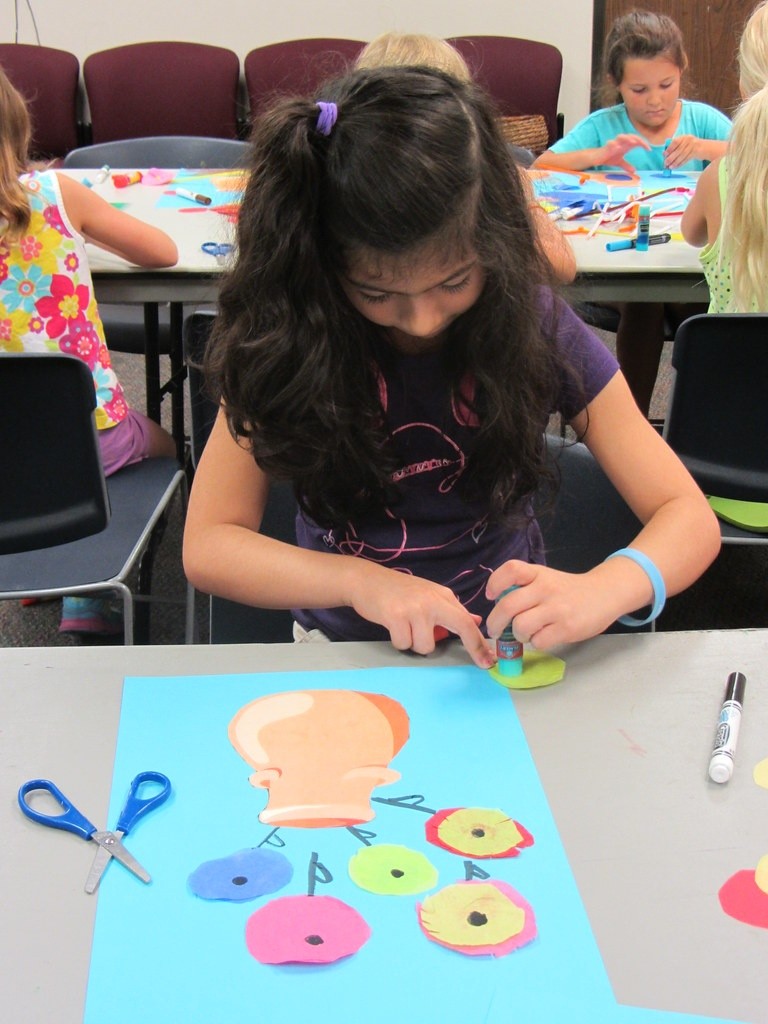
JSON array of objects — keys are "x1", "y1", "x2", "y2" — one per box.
[{"x1": 58, "y1": 596, "x2": 123, "y2": 635}]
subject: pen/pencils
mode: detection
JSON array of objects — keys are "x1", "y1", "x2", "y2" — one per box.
[{"x1": 586, "y1": 202, "x2": 610, "y2": 243}]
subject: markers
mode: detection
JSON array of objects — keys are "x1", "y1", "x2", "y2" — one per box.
[
  {"x1": 82, "y1": 179, "x2": 93, "y2": 188},
  {"x1": 708, "y1": 670, "x2": 748, "y2": 782},
  {"x1": 176, "y1": 188, "x2": 212, "y2": 205},
  {"x1": 94, "y1": 165, "x2": 110, "y2": 184},
  {"x1": 547, "y1": 200, "x2": 585, "y2": 222},
  {"x1": 606, "y1": 233, "x2": 672, "y2": 253}
]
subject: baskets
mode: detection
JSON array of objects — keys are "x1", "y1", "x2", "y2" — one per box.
[{"x1": 495, "y1": 114, "x2": 549, "y2": 151}]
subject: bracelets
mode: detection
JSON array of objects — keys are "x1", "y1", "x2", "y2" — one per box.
[{"x1": 604, "y1": 548, "x2": 666, "y2": 627}]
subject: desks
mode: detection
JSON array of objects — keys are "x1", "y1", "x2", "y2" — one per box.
[
  {"x1": 45, "y1": 169, "x2": 712, "y2": 463},
  {"x1": 0, "y1": 603, "x2": 768, "y2": 1020}
]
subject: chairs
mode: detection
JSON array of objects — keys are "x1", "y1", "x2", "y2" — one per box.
[
  {"x1": 0, "y1": 354, "x2": 186, "y2": 645},
  {"x1": 447, "y1": 36, "x2": 566, "y2": 151},
  {"x1": 244, "y1": 38, "x2": 371, "y2": 136},
  {"x1": 0, "y1": 42, "x2": 79, "y2": 163},
  {"x1": 82, "y1": 41, "x2": 241, "y2": 139},
  {"x1": 183, "y1": 309, "x2": 224, "y2": 645},
  {"x1": 649, "y1": 315, "x2": 766, "y2": 631}
]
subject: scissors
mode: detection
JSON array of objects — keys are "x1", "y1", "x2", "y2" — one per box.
[
  {"x1": 17, "y1": 771, "x2": 175, "y2": 894},
  {"x1": 201, "y1": 242, "x2": 233, "y2": 265}
]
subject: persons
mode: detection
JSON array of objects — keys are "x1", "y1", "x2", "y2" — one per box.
[
  {"x1": 356, "y1": 34, "x2": 576, "y2": 288},
  {"x1": 182, "y1": 64, "x2": 721, "y2": 668},
  {"x1": 528, "y1": 11, "x2": 737, "y2": 420},
  {"x1": 0, "y1": 69, "x2": 176, "y2": 646},
  {"x1": 679, "y1": 1, "x2": 768, "y2": 313}
]
